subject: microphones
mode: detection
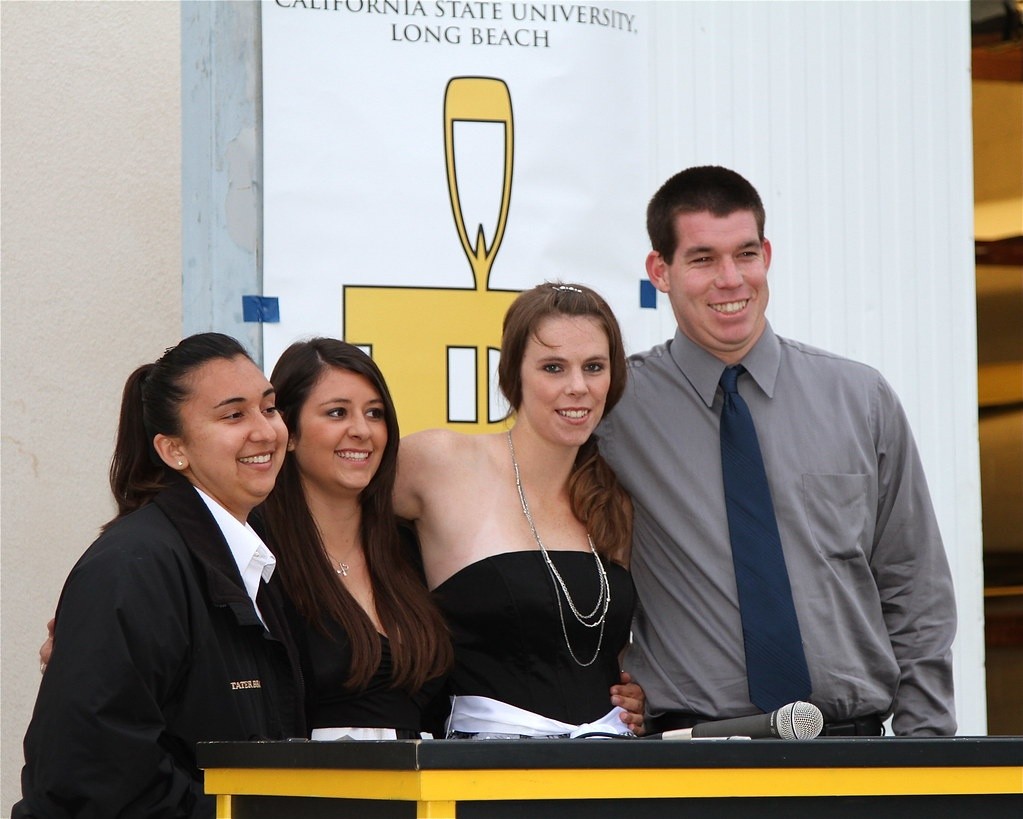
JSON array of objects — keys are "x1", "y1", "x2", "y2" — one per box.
[{"x1": 643, "y1": 701, "x2": 824, "y2": 741}]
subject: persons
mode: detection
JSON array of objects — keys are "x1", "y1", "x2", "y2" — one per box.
[
  {"x1": 41, "y1": 337, "x2": 645, "y2": 740},
  {"x1": 11, "y1": 333, "x2": 308, "y2": 819},
  {"x1": 591, "y1": 166, "x2": 958, "y2": 736},
  {"x1": 389, "y1": 283, "x2": 637, "y2": 740}
]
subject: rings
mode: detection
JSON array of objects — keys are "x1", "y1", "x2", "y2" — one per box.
[{"x1": 41, "y1": 664, "x2": 47, "y2": 674}]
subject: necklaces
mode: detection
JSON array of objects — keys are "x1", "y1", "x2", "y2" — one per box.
[
  {"x1": 508, "y1": 431, "x2": 610, "y2": 667},
  {"x1": 327, "y1": 552, "x2": 349, "y2": 576}
]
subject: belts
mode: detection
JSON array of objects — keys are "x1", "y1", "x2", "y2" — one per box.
[{"x1": 646, "y1": 709, "x2": 882, "y2": 735}]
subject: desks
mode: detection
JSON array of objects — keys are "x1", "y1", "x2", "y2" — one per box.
[{"x1": 193, "y1": 731, "x2": 1022, "y2": 819}]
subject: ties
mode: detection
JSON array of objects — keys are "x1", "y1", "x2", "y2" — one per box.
[{"x1": 720, "y1": 364, "x2": 814, "y2": 712}]
